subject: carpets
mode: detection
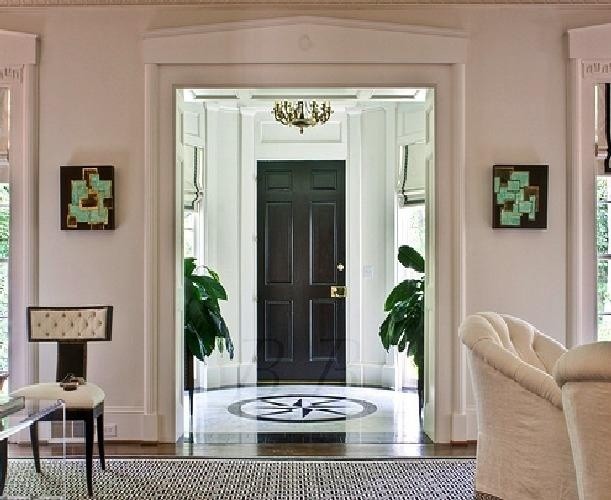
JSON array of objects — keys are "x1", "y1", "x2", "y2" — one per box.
[{"x1": 3, "y1": 454, "x2": 477, "y2": 500}]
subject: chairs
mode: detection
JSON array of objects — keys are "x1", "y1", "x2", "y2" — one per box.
[
  {"x1": 8, "y1": 304, "x2": 111, "y2": 499},
  {"x1": 459, "y1": 310, "x2": 611, "y2": 499}
]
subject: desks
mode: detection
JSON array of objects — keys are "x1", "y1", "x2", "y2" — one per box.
[{"x1": 0, "y1": 395, "x2": 66, "y2": 499}]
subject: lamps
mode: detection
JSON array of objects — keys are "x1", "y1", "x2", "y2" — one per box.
[{"x1": 270, "y1": 98, "x2": 334, "y2": 136}]
